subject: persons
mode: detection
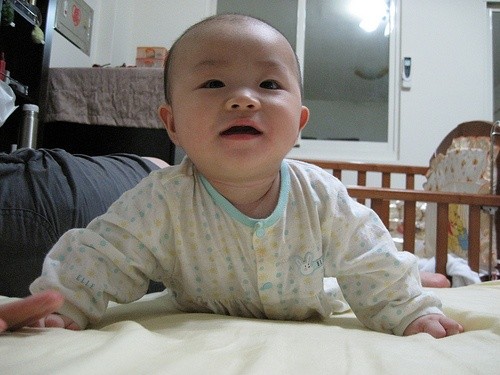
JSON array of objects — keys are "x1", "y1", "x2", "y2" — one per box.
[
  {"x1": 26, "y1": 11, "x2": 465, "y2": 339},
  {"x1": 1, "y1": 145, "x2": 452, "y2": 336}
]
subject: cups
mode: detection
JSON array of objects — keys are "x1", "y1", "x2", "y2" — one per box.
[{"x1": 17, "y1": 104, "x2": 40, "y2": 149}]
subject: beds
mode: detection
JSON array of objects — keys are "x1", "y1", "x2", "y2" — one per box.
[{"x1": 282, "y1": 121, "x2": 500, "y2": 287}]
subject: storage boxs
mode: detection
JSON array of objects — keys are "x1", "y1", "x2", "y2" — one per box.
[{"x1": 136, "y1": 46, "x2": 167, "y2": 67}]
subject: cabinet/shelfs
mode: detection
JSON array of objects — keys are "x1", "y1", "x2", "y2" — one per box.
[{"x1": 0, "y1": 0, "x2": 57, "y2": 121}]
